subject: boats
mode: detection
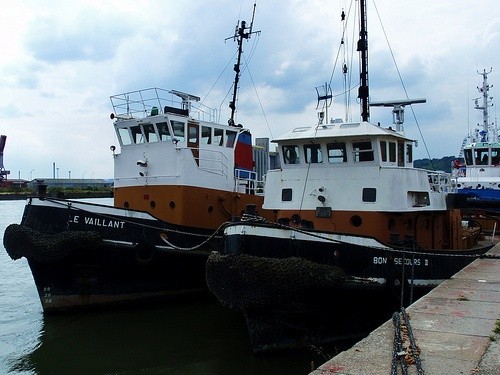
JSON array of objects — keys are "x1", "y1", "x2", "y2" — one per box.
[
  {"x1": 3, "y1": 0, "x2": 278, "y2": 318},
  {"x1": 446, "y1": 68, "x2": 500, "y2": 209},
  {"x1": 225, "y1": 1, "x2": 496, "y2": 280}
]
